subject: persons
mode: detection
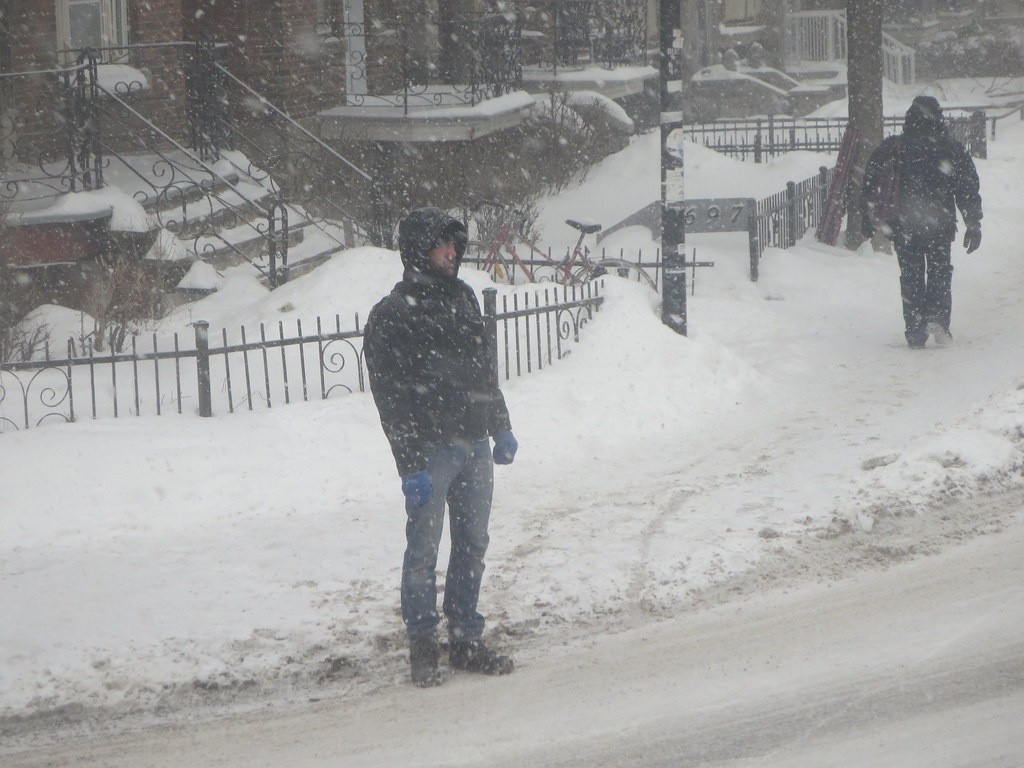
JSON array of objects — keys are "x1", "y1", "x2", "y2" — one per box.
[
  {"x1": 866, "y1": 95, "x2": 984, "y2": 346},
  {"x1": 361, "y1": 205, "x2": 519, "y2": 684}
]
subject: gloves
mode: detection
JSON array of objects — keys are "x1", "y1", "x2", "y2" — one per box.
[
  {"x1": 963, "y1": 220, "x2": 982, "y2": 254},
  {"x1": 402, "y1": 469, "x2": 432, "y2": 506},
  {"x1": 492, "y1": 430, "x2": 518, "y2": 465},
  {"x1": 861, "y1": 212, "x2": 879, "y2": 239}
]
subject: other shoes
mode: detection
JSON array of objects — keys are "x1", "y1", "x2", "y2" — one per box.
[
  {"x1": 409, "y1": 645, "x2": 440, "y2": 687},
  {"x1": 450, "y1": 642, "x2": 512, "y2": 674},
  {"x1": 927, "y1": 319, "x2": 956, "y2": 349},
  {"x1": 910, "y1": 330, "x2": 932, "y2": 349}
]
